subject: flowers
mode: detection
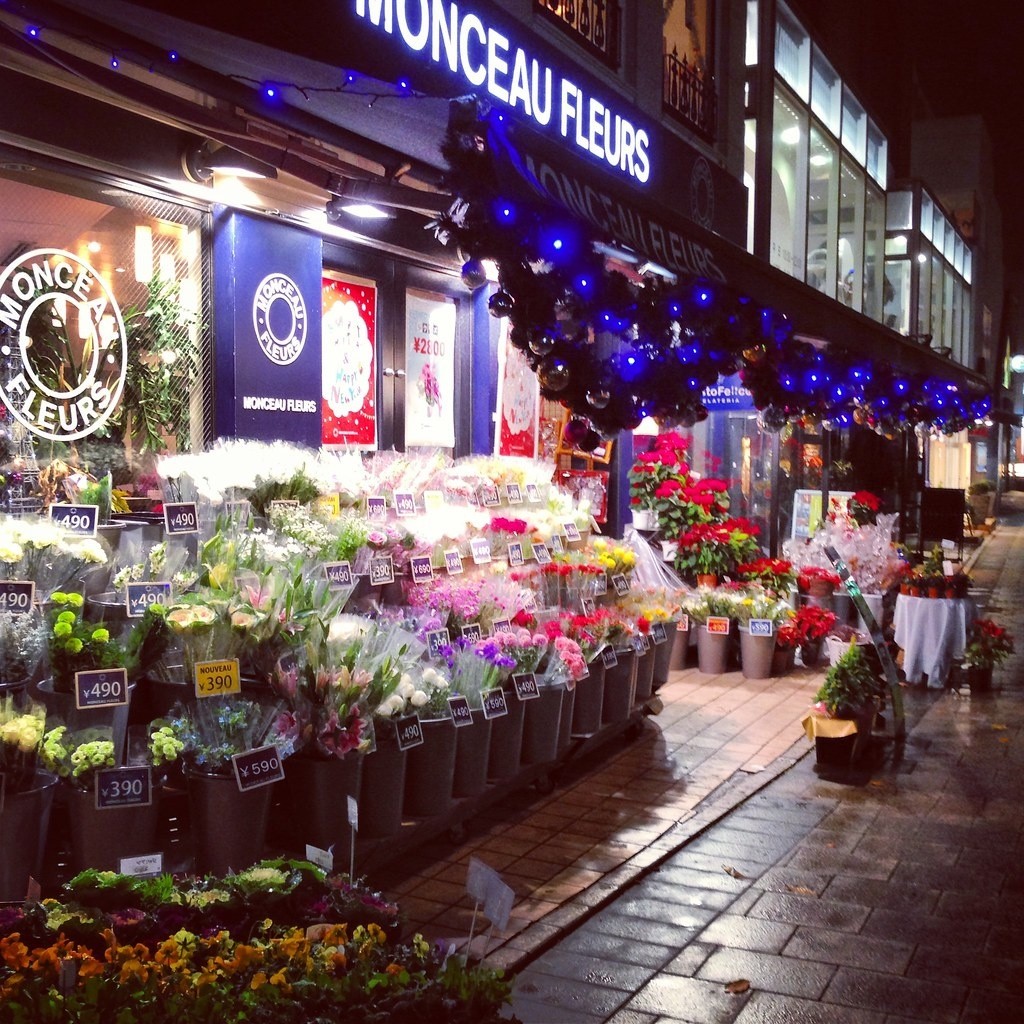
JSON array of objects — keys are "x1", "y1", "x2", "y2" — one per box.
[
  {"x1": 847, "y1": 489, "x2": 883, "y2": 524},
  {"x1": 0, "y1": 856, "x2": 513, "y2": 1024},
  {"x1": 963, "y1": 617, "x2": 1015, "y2": 666},
  {"x1": 909, "y1": 574, "x2": 973, "y2": 586},
  {"x1": 0, "y1": 434, "x2": 842, "y2": 789}
]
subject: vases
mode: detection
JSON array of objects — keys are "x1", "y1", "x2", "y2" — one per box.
[
  {"x1": 403, "y1": 712, "x2": 461, "y2": 813},
  {"x1": 487, "y1": 692, "x2": 524, "y2": 783},
  {"x1": 627, "y1": 509, "x2": 663, "y2": 532},
  {"x1": 804, "y1": 578, "x2": 836, "y2": 598},
  {"x1": 658, "y1": 540, "x2": 680, "y2": 563},
  {"x1": 669, "y1": 619, "x2": 691, "y2": 673},
  {"x1": 632, "y1": 632, "x2": 659, "y2": 699},
  {"x1": 573, "y1": 655, "x2": 603, "y2": 736},
  {"x1": 737, "y1": 626, "x2": 777, "y2": 679},
  {"x1": 824, "y1": 637, "x2": 869, "y2": 669},
  {"x1": 605, "y1": 646, "x2": 636, "y2": 722},
  {"x1": 0, "y1": 767, "x2": 61, "y2": 908},
  {"x1": 561, "y1": 680, "x2": 583, "y2": 745},
  {"x1": 360, "y1": 712, "x2": 407, "y2": 836},
  {"x1": 175, "y1": 764, "x2": 277, "y2": 883},
  {"x1": 900, "y1": 583, "x2": 970, "y2": 600},
  {"x1": 280, "y1": 750, "x2": 357, "y2": 855},
  {"x1": 452, "y1": 708, "x2": 496, "y2": 789},
  {"x1": 697, "y1": 574, "x2": 720, "y2": 593},
  {"x1": 698, "y1": 624, "x2": 729, "y2": 675},
  {"x1": 60, "y1": 771, "x2": 160, "y2": 886},
  {"x1": 652, "y1": 621, "x2": 678, "y2": 690},
  {"x1": 520, "y1": 681, "x2": 566, "y2": 763}
]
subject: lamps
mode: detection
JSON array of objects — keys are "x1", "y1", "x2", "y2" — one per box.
[
  {"x1": 177, "y1": 144, "x2": 278, "y2": 186},
  {"x1": 913, "y1": 334, "x2": 934, "y2": 347},
  {"x1": 325, "y1": 198, "x2": 397, "y2": 225},
  {"x1": 929, "y1": 346, "x2": 952, "y2": 358}
]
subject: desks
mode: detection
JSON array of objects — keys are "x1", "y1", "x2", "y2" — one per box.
[{"x1": 892, "y1": 593, "x2": 978, "y2": 689}]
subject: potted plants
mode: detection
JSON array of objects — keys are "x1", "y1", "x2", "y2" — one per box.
[
  {"x1": 966, "y1": 482, "x2": 990, "y2": 525},
  {"x1": 977, "y1": 479, "x2": 997, "y2": 516},
  {"x1": 812, "y1": 630, "x2": 883, "y2": 722}
]
